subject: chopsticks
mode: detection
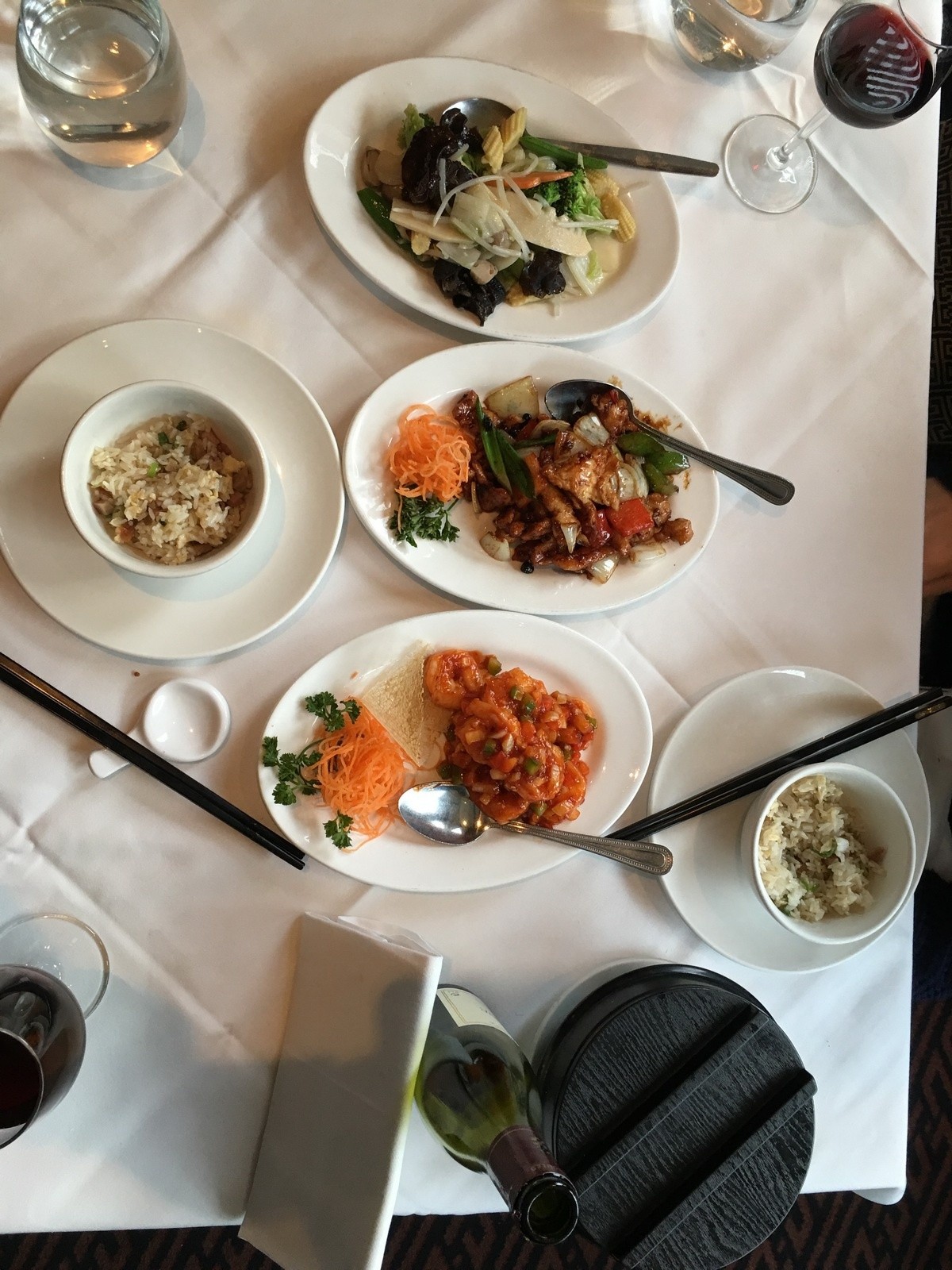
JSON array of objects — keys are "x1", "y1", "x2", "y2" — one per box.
[
  {"x1": 603, "y1": 681, "x2": 951, "y2": 846},
  {"x1": 0, "y1": 650, "x2": 309, "y2": 870}
]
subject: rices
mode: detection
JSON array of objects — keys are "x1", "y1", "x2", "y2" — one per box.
[
  {"x1": 753, "y1": 777, "x2": 886, "y2": 926},
  {"x1": 85, "y1": 414, "x2": 253, "y2": 567}
]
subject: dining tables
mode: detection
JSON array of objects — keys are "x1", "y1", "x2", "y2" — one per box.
[{"x1": 0, "y1": 3, "x2": 944, "y2": 1232}]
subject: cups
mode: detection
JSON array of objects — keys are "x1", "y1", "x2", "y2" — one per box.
[
  {"x1": 16, "y1": 3, "x2": 187, "y2": 174},
  {"x1": 664, "y1": 0, "x2": 816, "y2": 74}
]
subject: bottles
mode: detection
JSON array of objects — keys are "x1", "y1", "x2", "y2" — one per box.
[{"x1": 407, "y1": 981, "x2": 580, "y2": 1248}]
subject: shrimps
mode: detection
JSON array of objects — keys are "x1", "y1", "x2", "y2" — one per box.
[{"x1": 424, "y1": 649, "x2": 596, "y2": 829}]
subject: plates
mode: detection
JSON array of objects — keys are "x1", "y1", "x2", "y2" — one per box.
[
  {"x1": 305, "y1": 56, "x2": 678, "y2": 340},
  {"x1": 645, "y1": 664, "x2": 931, "y2": 975},
  {"x1": 1, "y1": 316, "x2": 347, "y2": 662},
  {"x1": 531, "y1": 956, "x2": 674, "y2": 1072},
  {"x1": 257, "y1": 608, "x2": 652, "y2": 896},
  {"x1": 344, "y1": 340, "x2": 720, "y2": 615}
]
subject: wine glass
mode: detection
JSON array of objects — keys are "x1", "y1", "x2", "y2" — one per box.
[
  {"x1": 2, "y1": 910, "x2": 110, "y2": 1150},
  {"x1": 720, "y1": 0, "x2": 949, "y2": 215}
]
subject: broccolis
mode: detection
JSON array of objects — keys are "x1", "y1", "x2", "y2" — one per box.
[{"x1": 522, "y1": 161, "x2": 618, "y2": 235}]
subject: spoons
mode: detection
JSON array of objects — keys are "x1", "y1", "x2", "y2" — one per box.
[
  {"x1": 397, "y1": 783, "x2": 673, "y2": 878},
  {"x1": 443, "y1": 101, "x2": 719, "y2": 185},
  {"x1": 543, "y1": 379, "x2": 795, "y2": 510}
]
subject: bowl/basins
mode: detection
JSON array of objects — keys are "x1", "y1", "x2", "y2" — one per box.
[
  {"x1": 736, "y1": 762, "x2": 915, "y2": 945},
  {"x1": 61, "y1": 378, "x2": 271, "y2": 579}
]
